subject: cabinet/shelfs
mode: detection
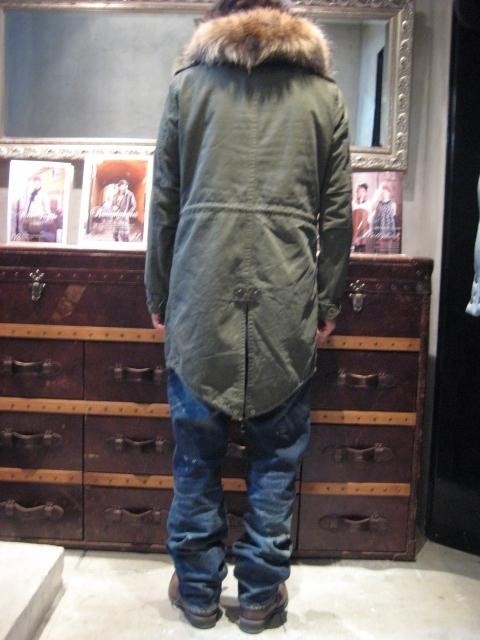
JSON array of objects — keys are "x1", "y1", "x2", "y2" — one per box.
[{"x1": 1, "y1": 246, "x2": 434, "y2": 563}]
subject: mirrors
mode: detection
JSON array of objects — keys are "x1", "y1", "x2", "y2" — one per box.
[{"x1": 1, "y1": 0, "x2": 411, "y2": 170}]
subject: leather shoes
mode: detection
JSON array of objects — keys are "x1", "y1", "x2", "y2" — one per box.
[
  {"x1": 168, "y1": 571, "x2": 218, "y2": 629},
  {"x1": 238, "y1": 582, "x2": 288, "y2": 633}
]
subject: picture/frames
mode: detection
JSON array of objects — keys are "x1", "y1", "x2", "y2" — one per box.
[
  {"x1": 77, "y1": 148, "x2": 156, "y2": 250},
  {"x1": 6, "y1": 156, "x2": 72, "y2": 246}
]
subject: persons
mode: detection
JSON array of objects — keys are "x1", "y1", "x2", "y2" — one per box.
[
  {"x1": 18, "y1": 176, "x2": 54, "y2": 221},
  {"x1": 353, "y1": 181, "x2": 402, "y2": 254},
  {"x1": 88, "y1": 180, "x2": 136, "y2": 240},
  {"x1": 143, "y1": 0, "x2": 351, "y2": 633}
]
